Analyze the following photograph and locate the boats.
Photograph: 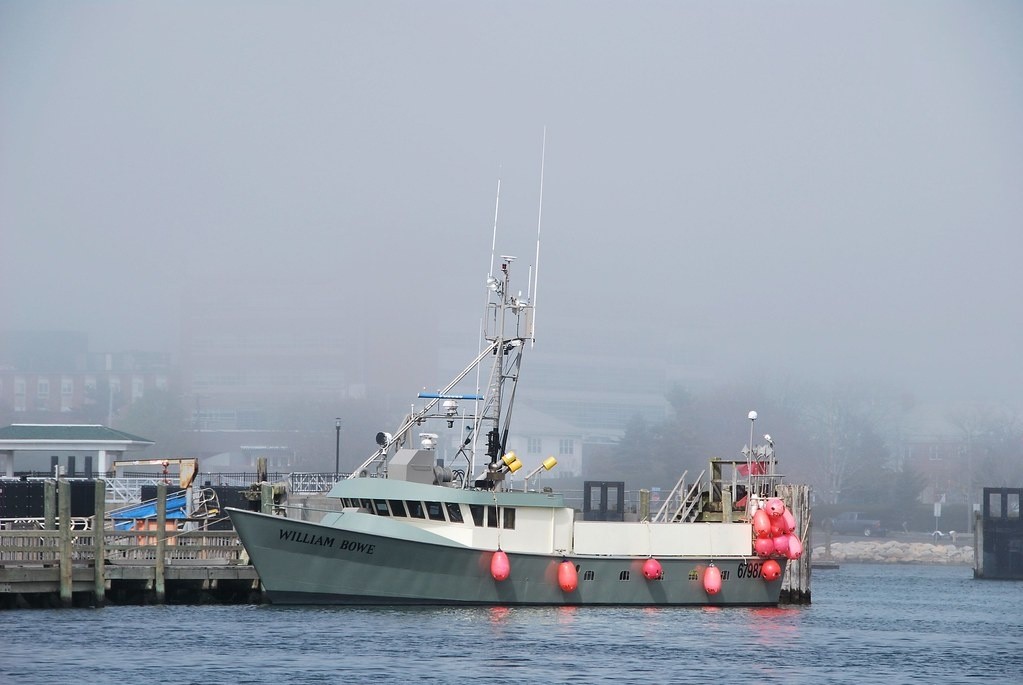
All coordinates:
[223,121,803,608]
[71,487,253,567]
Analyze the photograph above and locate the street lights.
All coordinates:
[335,416,342,483]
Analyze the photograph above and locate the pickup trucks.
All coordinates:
[821,510,887,537]
